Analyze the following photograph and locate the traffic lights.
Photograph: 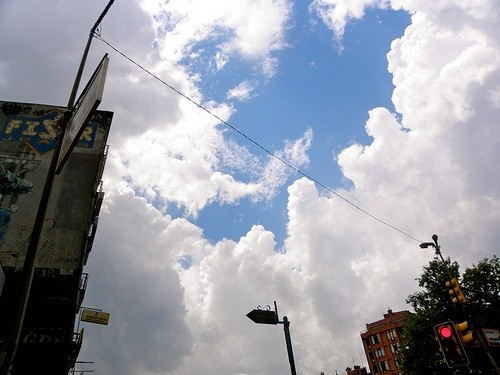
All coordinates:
[434,321,470,369]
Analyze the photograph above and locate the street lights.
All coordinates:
[419,233,499,375]
